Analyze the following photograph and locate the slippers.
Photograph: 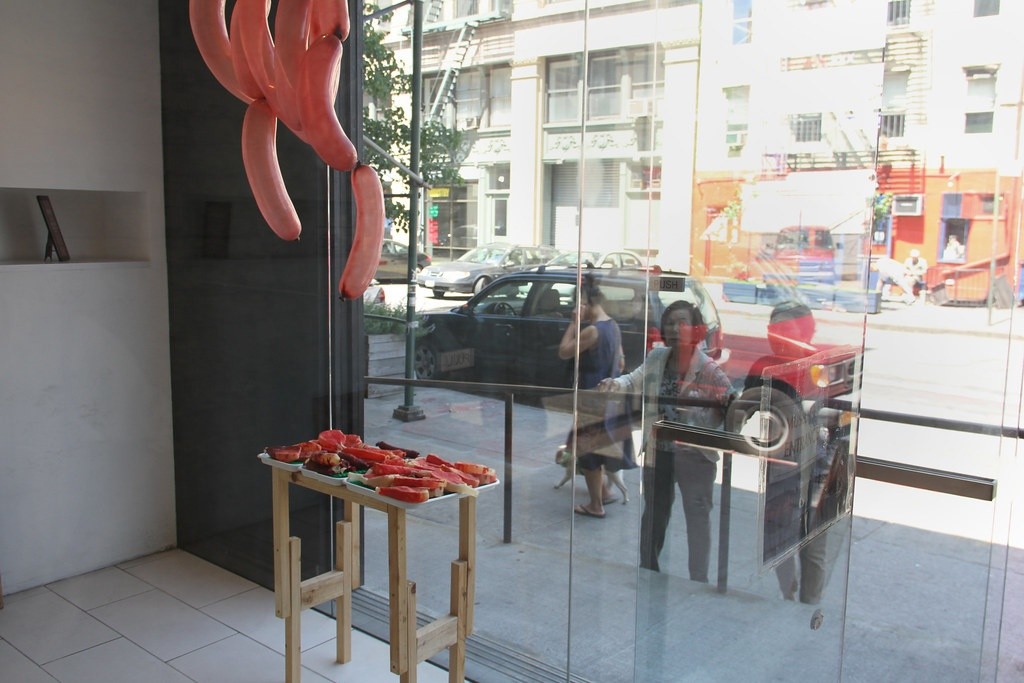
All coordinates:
[574,503,606,518]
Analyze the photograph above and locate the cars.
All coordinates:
[520,250,643,304]
[423,243,564,300]
[371,237,433,283]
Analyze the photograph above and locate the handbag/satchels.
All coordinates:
[571,383,615,420]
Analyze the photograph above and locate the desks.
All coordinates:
[268,454,479,683]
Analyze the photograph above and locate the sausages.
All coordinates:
[189,0,386,303]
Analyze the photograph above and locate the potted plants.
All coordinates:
[834,284,881,315]
[362,292,435,399]
[757,282,795,306]
[796,280,834,311]
[868,192,892,241]
[721,278,761,304]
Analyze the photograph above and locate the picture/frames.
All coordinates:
[35,194,69,262]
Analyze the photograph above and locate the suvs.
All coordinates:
[416,261,723,411]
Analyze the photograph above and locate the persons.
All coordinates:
[942,234,965,262]
[742,299,841,605]
[596,300,733,584]
[904,249,927,290]
[558,282,625,518]
